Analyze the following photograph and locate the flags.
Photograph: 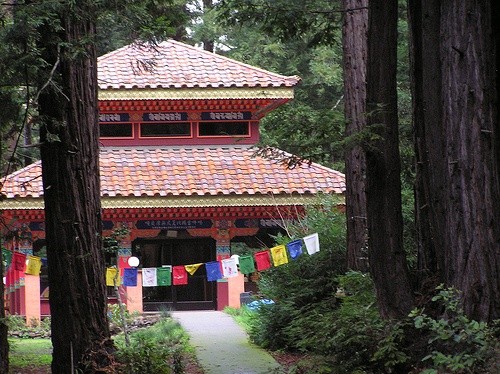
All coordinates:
[0,232,332,287]
